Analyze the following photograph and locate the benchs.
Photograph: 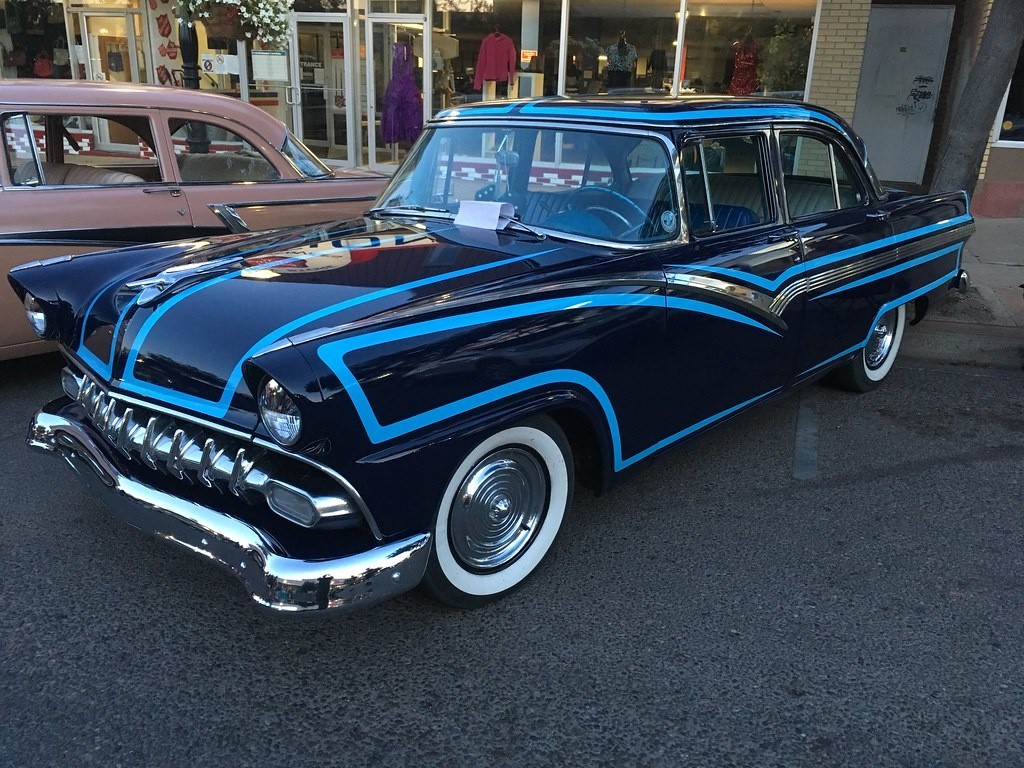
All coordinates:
[625,174,855,222]
[180,153,276,183]
[13,161,144,185]
[512,190,761,242]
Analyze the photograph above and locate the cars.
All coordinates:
[301,87,383,147]
[0,77,460,362]
[7,88,977,624]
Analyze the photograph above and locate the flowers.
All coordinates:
[171,0,295,53]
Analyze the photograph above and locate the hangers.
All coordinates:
[482,24,513,42]
